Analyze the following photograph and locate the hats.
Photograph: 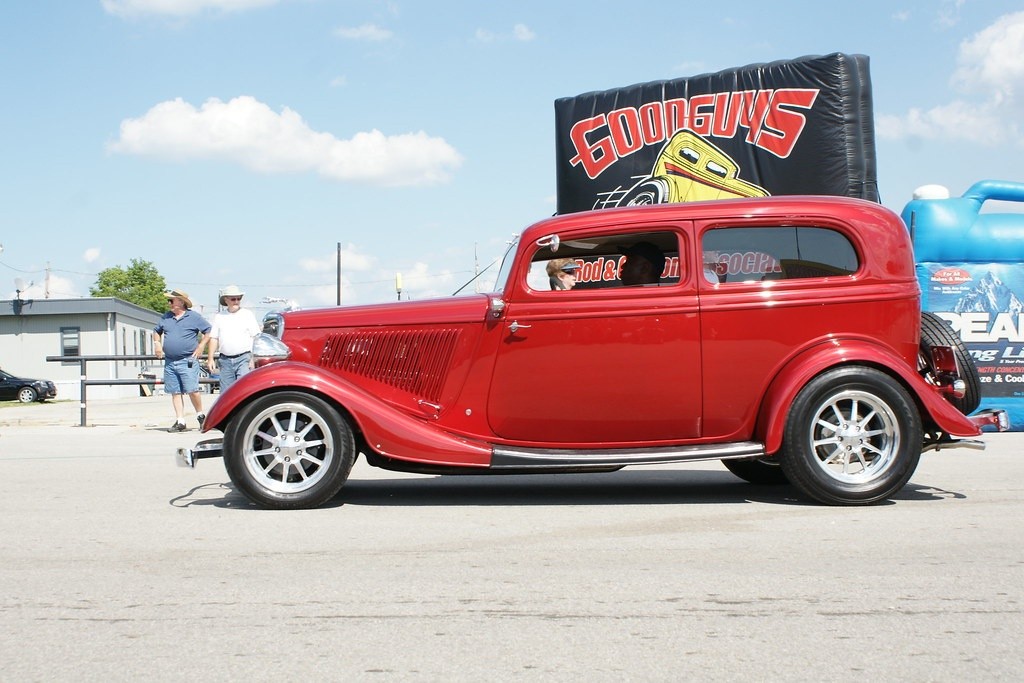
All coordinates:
[164,290,192,308]
[547,258,581,277]
[219,287,243,306]
[617,241,665,272]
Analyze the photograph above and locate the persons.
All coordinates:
[154,289,212,432]
[546,258,580,290]
[207,286,261,395]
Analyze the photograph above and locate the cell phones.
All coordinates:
[188,360,193,368]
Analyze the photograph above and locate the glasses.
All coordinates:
[167,298,182,304]
[556,269,575,275]
[226,297,241,302]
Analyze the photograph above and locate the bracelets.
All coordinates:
[154,341,160,343]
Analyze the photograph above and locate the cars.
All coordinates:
[176,193,1011,508]
[208,367,221,394]
[0,369,58,404]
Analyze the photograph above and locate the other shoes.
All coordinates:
[167,421,186,433]
[198,415,206,431]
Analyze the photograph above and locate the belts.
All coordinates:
[221,351,250,358]
